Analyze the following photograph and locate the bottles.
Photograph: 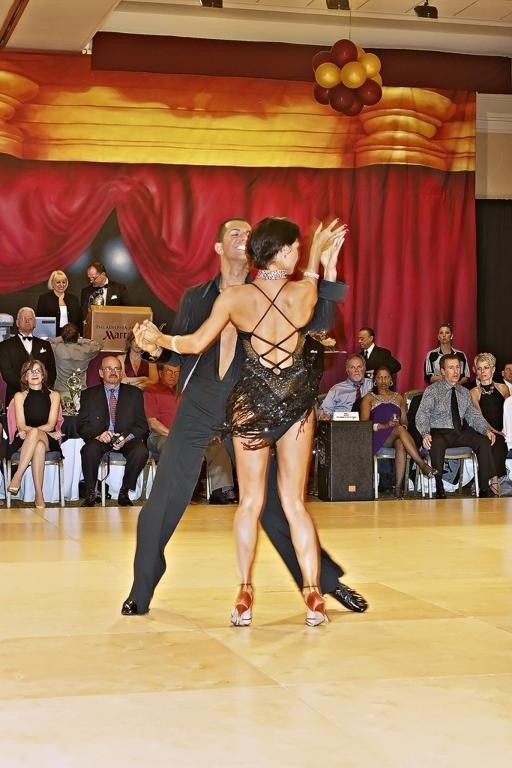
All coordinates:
[94,292,105,306]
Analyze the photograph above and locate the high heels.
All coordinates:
[7,478,21,494]
[229,580,255,629]
[34,493,47,511]
[419,461,440,480]
[298,582,331,629]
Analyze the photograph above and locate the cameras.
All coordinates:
[109,433,127,448]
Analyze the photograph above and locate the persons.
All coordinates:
[1,258,239,508]
[320,322,512,499]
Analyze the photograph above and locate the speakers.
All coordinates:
[309,420,373,501]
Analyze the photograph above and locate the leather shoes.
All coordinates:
[208,492,239,505]
[328,581,370,614]
[80,488,98,508]
[117,488,134,507]
[120,584,151,616]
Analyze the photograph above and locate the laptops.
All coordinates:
[34,317,56,337]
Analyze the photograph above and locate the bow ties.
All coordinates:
[22,335,34,342]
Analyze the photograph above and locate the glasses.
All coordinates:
[103,366,122,373]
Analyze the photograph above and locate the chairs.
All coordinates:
[0,394,217,507]
[304,382,484,497]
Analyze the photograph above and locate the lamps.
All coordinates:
[325,0,350,11]
[413,0,438,17]
[200,0,224,8]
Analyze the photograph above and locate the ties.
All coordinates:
[450,386,465,437]
